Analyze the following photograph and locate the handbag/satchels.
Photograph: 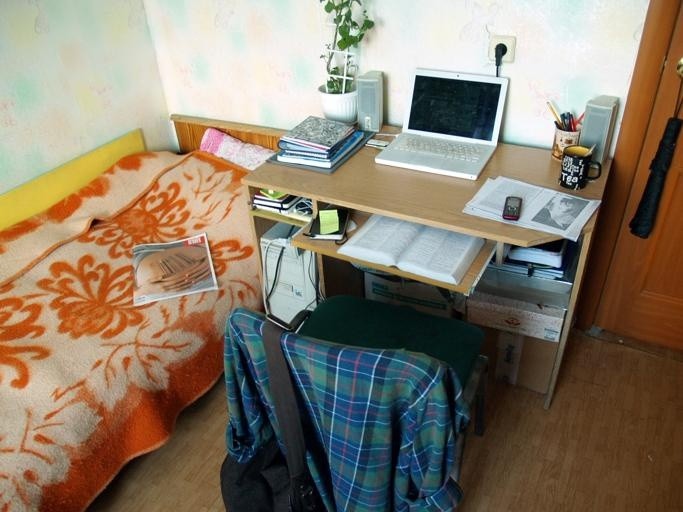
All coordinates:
[220,314,318,512]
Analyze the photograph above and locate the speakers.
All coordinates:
[579,95,620,168]
[356,71,384,133]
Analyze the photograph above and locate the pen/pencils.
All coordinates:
[545,101,584,132]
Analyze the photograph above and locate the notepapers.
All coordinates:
[318,210,338,234]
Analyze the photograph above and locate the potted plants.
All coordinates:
[319,0,374,124]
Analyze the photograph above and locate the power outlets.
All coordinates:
[488,36,516,63]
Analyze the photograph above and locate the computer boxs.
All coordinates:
[259,222,317,326]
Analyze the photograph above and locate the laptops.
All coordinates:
[375,69,509,181]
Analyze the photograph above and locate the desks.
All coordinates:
[240,125,613,411]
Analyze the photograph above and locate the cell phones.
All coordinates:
[503,196,522,220]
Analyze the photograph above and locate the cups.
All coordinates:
[558,145,602,191]
[551,123,582,162]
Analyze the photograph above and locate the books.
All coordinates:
[338,214,487,286]
[309,204,350,241]
[131,232,219,307]
[267,115,377,174]
[252,188,313,223]
[488,247,576,279]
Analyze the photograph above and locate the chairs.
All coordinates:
[226,295,489,483]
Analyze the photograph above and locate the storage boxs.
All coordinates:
[494,331,559,395]
[465,291,566,343]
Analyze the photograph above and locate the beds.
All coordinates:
[1,114,292,511]
